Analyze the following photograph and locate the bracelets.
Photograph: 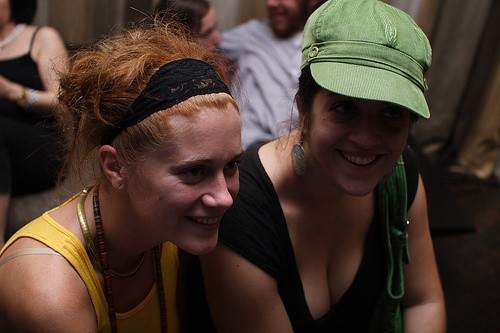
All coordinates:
[28,88,38,108]
[17,86,28,107]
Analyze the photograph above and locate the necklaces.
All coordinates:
[0,23,26,49]
[92,183,168,333]
[76,185,149,279]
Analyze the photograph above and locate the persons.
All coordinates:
[157,0,222,46]
[0,0,70,250]
[0,24,248,333]
[200,0,446,333]
[216,0,325,150]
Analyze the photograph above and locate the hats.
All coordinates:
[301,0,431,118]
